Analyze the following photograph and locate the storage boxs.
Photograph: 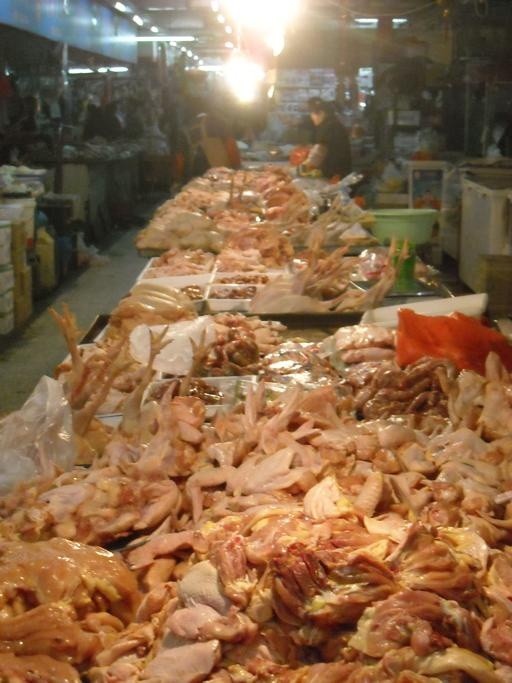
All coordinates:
[0,192,81,337]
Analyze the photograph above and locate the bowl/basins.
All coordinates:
[362,207,438,244]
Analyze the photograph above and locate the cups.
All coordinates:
[390,237,418,291]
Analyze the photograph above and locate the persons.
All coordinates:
[173,96,352,197]
